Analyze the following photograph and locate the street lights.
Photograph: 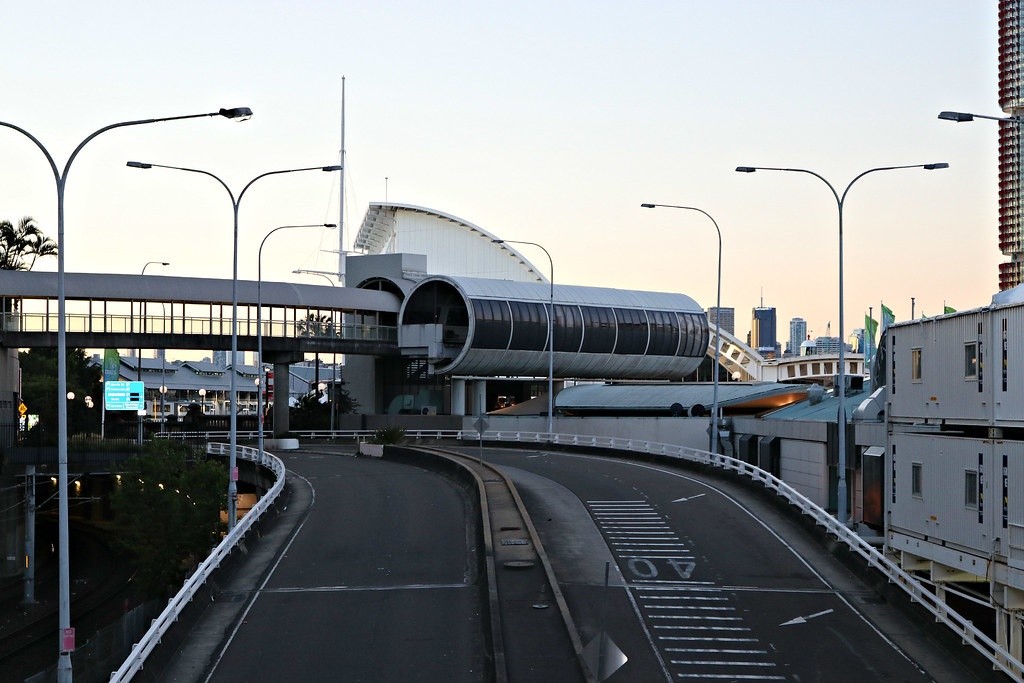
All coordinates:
[85,395,93,437]
[640,202,722,467]
[198,388,207,415]
[292,270,346,335]
[318,382,325,398]
[0,105,253,682]
[159,385,167,420]
[254,376,264,416]
[128,161,345,535]
[138,261,171,448]
[257,222,335,465]
[492,239,554,441]
[66,392,75,436]
[264,365,270,408]
[734,161,950,527]
